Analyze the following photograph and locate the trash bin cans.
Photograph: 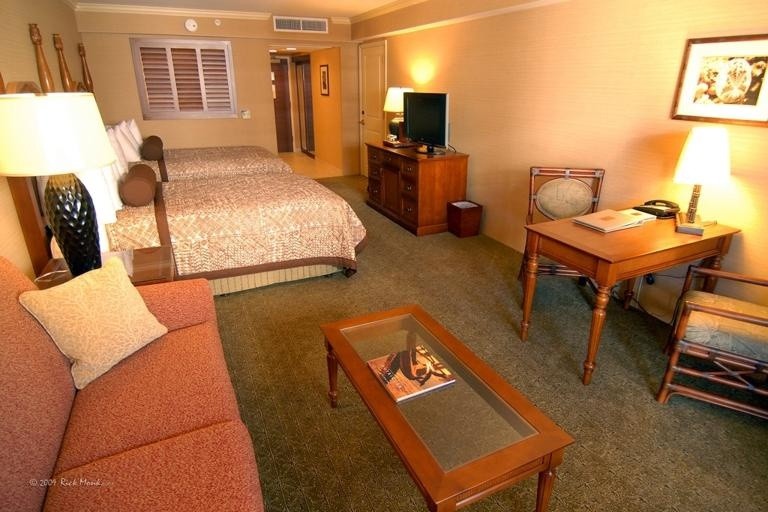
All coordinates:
[447,199,483,238]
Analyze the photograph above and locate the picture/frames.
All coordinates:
[319,64,330,97]
[667,33,768,132]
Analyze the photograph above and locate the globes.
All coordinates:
[524,177,593,276]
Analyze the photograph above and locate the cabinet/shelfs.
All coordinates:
[363,140,471,238]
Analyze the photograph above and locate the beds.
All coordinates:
[128,143,295,183]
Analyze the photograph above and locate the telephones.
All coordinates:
[633,199,680,217]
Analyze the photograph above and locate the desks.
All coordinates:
[519,208,742,388]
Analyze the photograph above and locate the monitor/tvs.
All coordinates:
[404,92,449,155]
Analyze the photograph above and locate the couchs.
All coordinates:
[0,250,267,511]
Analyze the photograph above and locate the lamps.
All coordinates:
[382,87,415,140]
[0,91,122,277]
[671,123,733,238]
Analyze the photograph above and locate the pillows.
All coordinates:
[15,255,171,395]
[72,117,165,256]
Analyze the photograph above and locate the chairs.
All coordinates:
[653,264,767,420]
[517,164,607,344]
[50,173,370,296]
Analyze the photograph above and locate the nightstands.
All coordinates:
[34,243,179,287]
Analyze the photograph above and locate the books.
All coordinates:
[620,209,657,223]
[367,344,456,404]
[573,207,643,233]
